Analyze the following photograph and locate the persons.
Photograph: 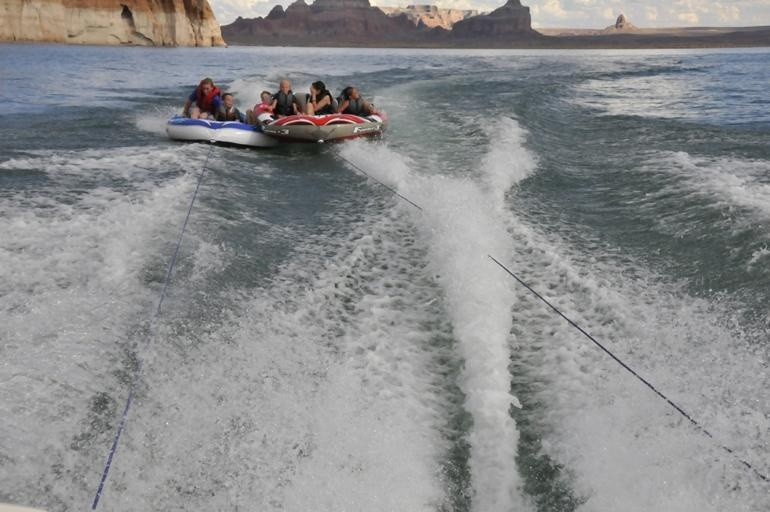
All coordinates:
[305,80,336,117]
[212,92,242,123]
[182,76,221,121]
[266,79,299,120]
[244,91,279,126]
[337,86,371,115]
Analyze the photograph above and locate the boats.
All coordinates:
[166,113,278,148]
[253,103,388,142]
